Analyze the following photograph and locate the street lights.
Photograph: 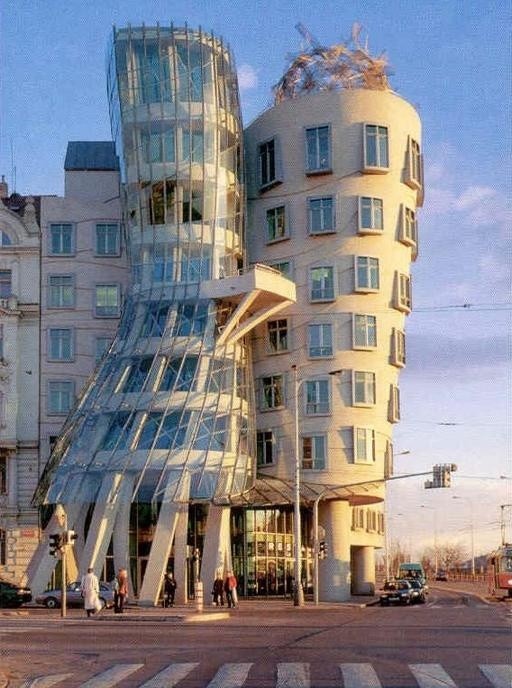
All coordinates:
[291,364,343,607]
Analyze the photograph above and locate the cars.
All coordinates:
[0,581,32,609]
[35,581,114,609]
[380,562,429,607]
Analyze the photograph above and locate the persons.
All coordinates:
[224,570,237,609]
[385,569,422,591]
[164,570,177,607]
[80,567,102,618]
[212,572,223,606]
[161,574,171,608]
[113,567,130,613]
